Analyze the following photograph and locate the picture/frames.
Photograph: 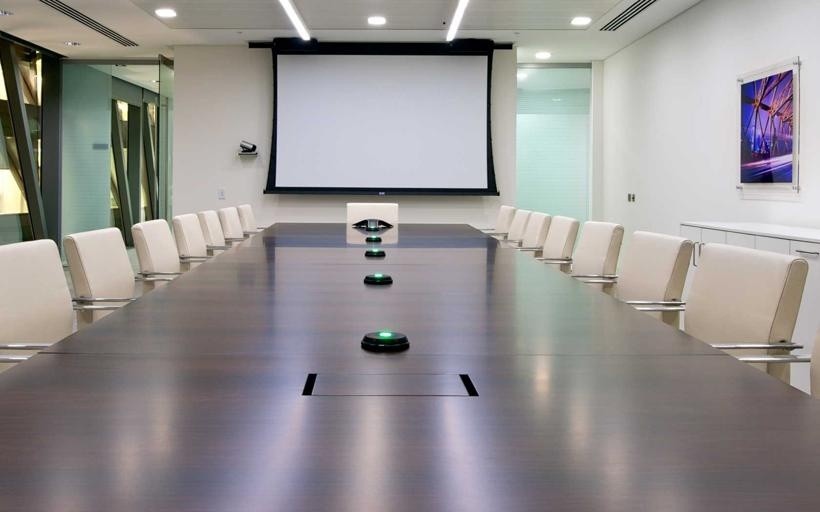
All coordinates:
[736,56,799,194]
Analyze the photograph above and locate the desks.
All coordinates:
[0,223,819,511]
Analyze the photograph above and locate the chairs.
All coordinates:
[63,227,172,330]
[516,216,579,273]
[133,218,208,295]
[478,205,516,238]
[740,306,820,397]
[1,238,121,357]
[171,212,227,271]
[238,203,268,236]
[219,206,256,246]
[499,212,551,257]
[487,209,530,246]
[345,202,399,222]
[543,221,626,298]
[196,209,243,256]
[638,241,809,384]
[582,229,695,330]
